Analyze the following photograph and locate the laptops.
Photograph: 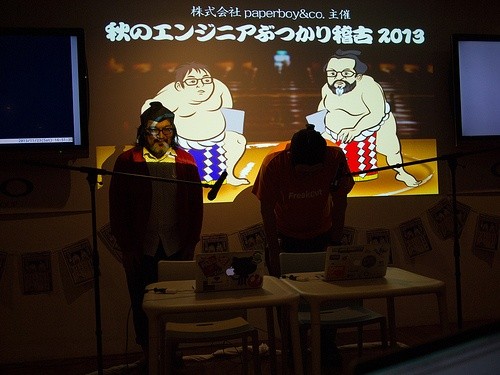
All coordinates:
[315,245,388,282]
[190,250,265,293]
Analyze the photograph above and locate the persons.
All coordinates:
[108,103,204,357]
[250,125,355,275]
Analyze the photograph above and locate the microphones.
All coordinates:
[207,171,228,201]
[330,157,349,193]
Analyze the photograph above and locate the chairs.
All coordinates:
[280,251,387,372]
[160,259,262,375]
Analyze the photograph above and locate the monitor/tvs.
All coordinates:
[0,27,89,151]
[448,32,500,145]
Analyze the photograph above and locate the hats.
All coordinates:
[290,123,328,165]
[141,101,174,123]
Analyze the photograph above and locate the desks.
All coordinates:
[142,275,303,375]
[280,266,445,375]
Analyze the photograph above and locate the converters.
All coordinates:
[296,276,309,281]
[165,288,177,294]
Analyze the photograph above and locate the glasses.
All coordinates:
[143,126,174,137]
[180,75,213,86]
[326,69,356,78]
